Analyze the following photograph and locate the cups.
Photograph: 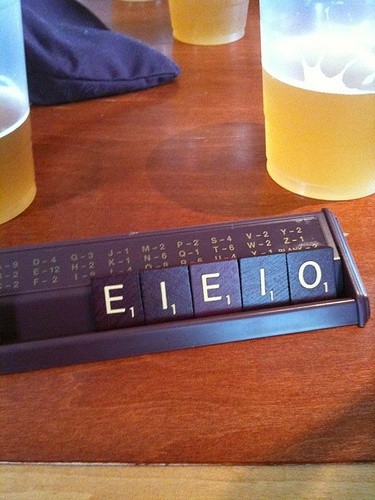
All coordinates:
[257,0,375,201]
[1,0,39,225]
[167,0,252,46]
